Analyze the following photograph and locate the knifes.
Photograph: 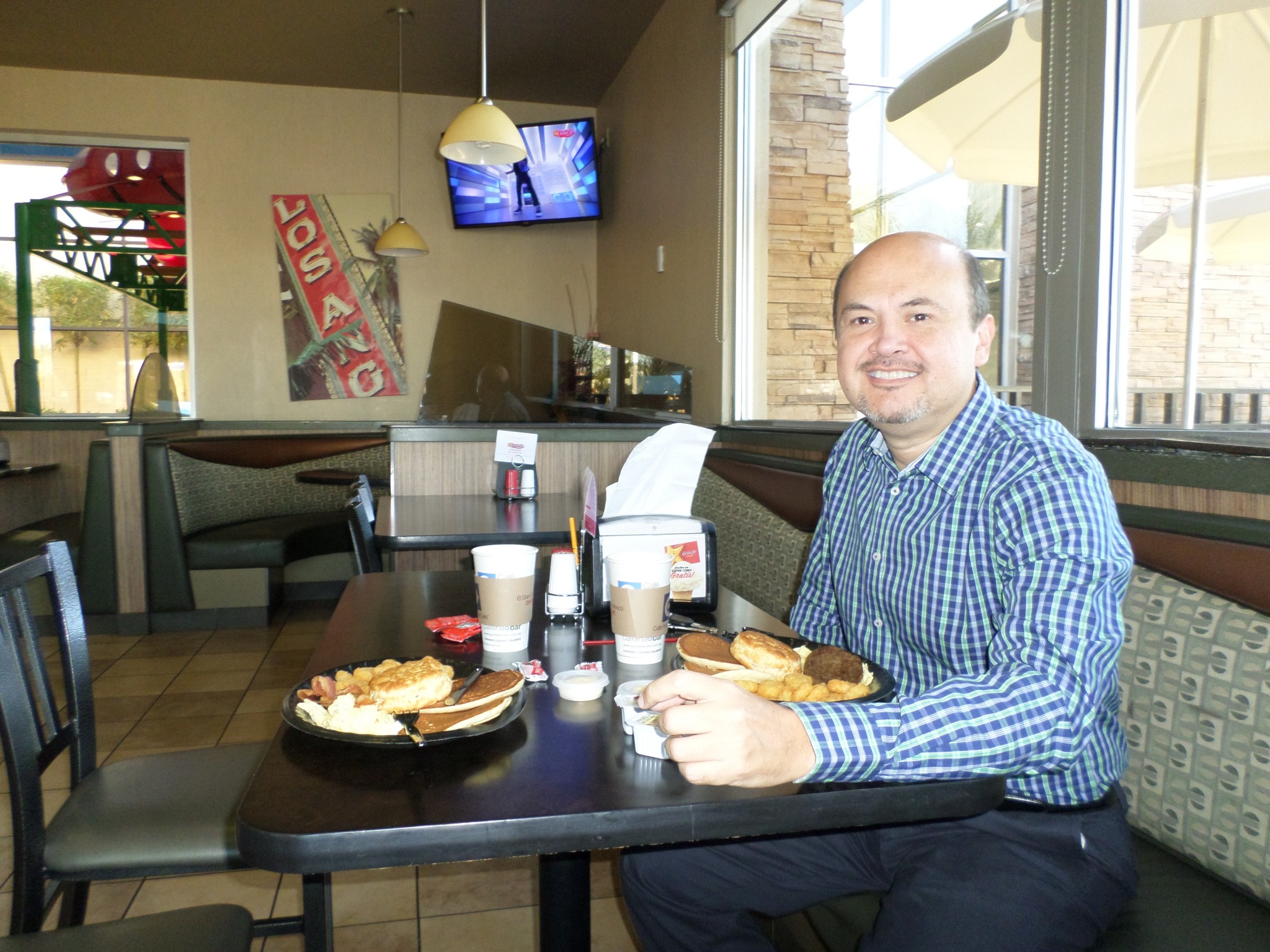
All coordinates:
[444,667,483,705]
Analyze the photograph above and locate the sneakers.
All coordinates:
[513,208,523,215]
[535,206,542,217]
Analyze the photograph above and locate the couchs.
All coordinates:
[145,431,391,633]
[0,436,118,617]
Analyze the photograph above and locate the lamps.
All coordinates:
[437,0,528,166]
[372,8,429,259]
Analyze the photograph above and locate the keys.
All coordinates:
[665,612,719,633]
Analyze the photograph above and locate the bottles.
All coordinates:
[505,470,518,498]
[548,547,578,615]
[520,470,534,497]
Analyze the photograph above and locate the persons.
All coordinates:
[475,364,532,422]
[505,157,542,218]
[617,228,1138,952]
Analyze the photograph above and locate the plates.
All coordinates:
[671,636,896,703]
[280,656,528,747]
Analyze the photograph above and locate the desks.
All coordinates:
[0,462,58,483]
[372,492,602,570]
[293,466,392,532]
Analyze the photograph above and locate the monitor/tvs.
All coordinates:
[441,118,603,229]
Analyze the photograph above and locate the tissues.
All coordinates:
[582,423,720,617]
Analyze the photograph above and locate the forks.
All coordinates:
[393,710,428,746]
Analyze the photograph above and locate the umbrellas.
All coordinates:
[881,0,1270,432]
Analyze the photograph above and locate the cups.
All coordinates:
[602,551,675,664]
[471,545,539,652]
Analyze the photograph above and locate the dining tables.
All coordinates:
[234,569,1010,952]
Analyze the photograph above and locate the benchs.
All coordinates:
[688,444,1270,951]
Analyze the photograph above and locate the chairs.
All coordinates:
[0,627,257,952]
[346,474,377,527]
[0,539,336,952]
[338,490,381,575]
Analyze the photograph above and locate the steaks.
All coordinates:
[803,644,864,686]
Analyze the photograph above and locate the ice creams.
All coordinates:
[669,563,702,601]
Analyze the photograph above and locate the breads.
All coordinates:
[369,656,525,735]
[675,631,803,683]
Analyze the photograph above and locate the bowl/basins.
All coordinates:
[552,670,610,701]
[616,680,656,735]
